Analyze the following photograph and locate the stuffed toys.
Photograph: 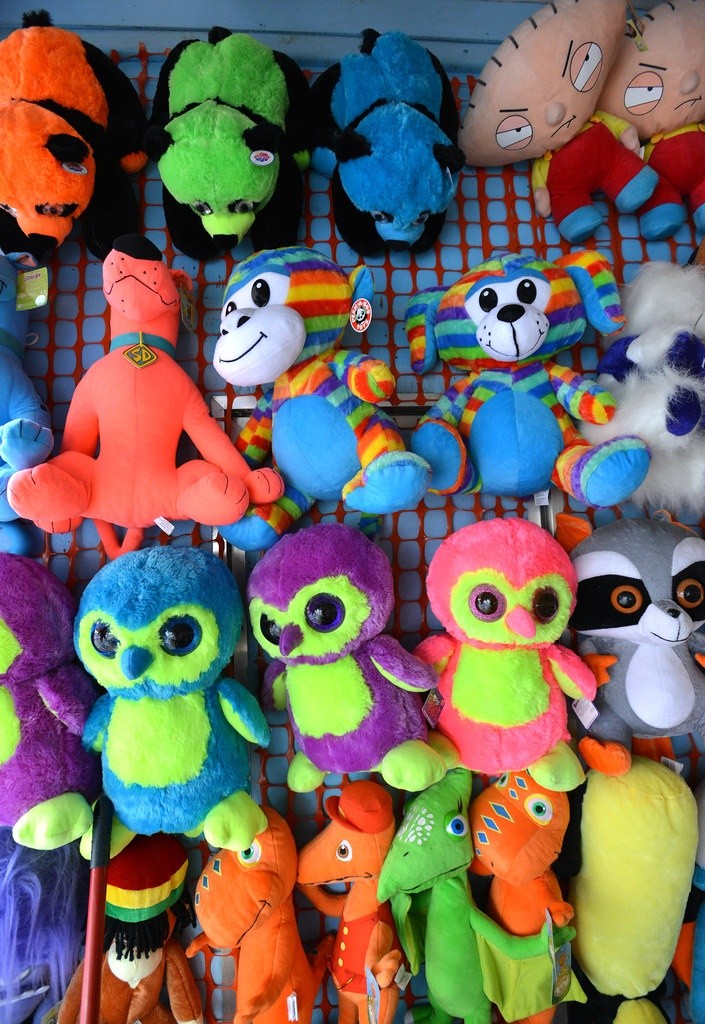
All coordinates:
[552,507,704,778]
[453,0,661,247]
[413,515,601,788]
[574,258,705,527]
[307,28,469,259]
[210,247,437,554]
[6,231,287,565]
[0,753,705,1024]
[403,252,654,509]
[68,541,276,860]
[598,0,704,239]
[144,22,321,263]
[0,8,156,260]
[241,521,448,798]
[1,551,96,854]
[0,246,53,555]
